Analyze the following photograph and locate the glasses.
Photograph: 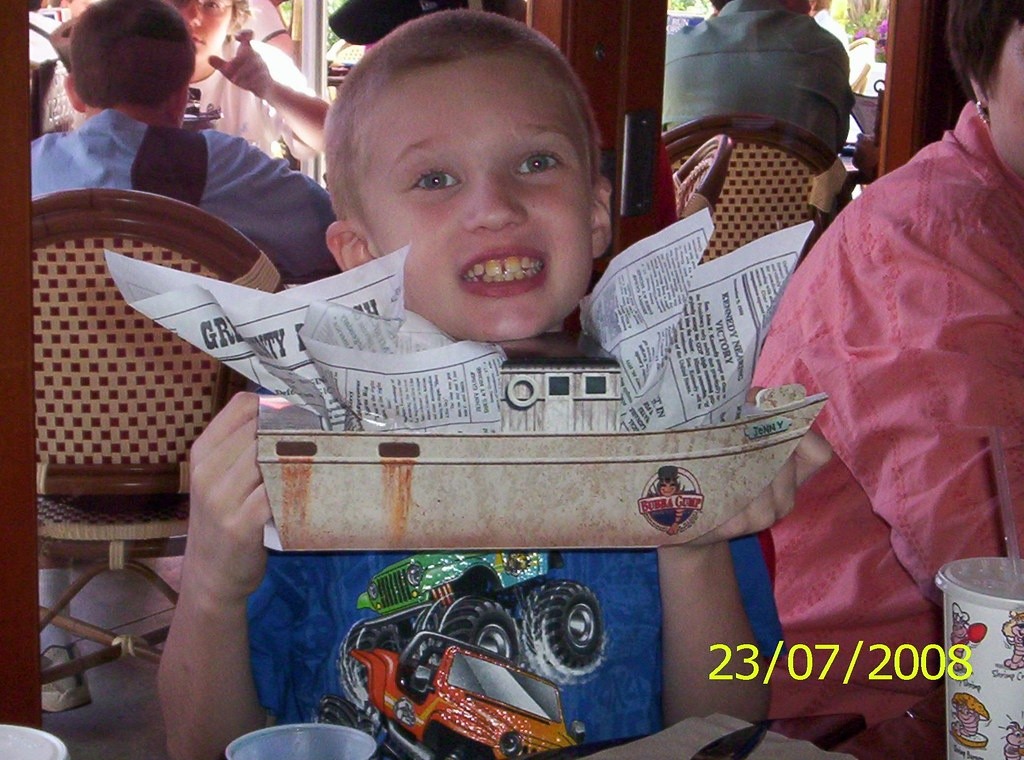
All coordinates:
[175,0,232,16]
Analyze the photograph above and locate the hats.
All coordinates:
[328,0,513,45]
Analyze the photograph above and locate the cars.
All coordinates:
[324,38,366,87]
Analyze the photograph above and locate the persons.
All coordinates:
[27,1,103,141]
[741,0,1022,759]
[154,9,790,759]
[661,1,856,157]
[807,0,850,55]
[241,0,296,58]
[31,0,344,713]
[327,0,510,61]
[156,0,334,164]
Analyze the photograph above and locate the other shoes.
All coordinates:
[40,643,90,714]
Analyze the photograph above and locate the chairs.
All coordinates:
[664,114,856,267]
[30,185,286,662]
